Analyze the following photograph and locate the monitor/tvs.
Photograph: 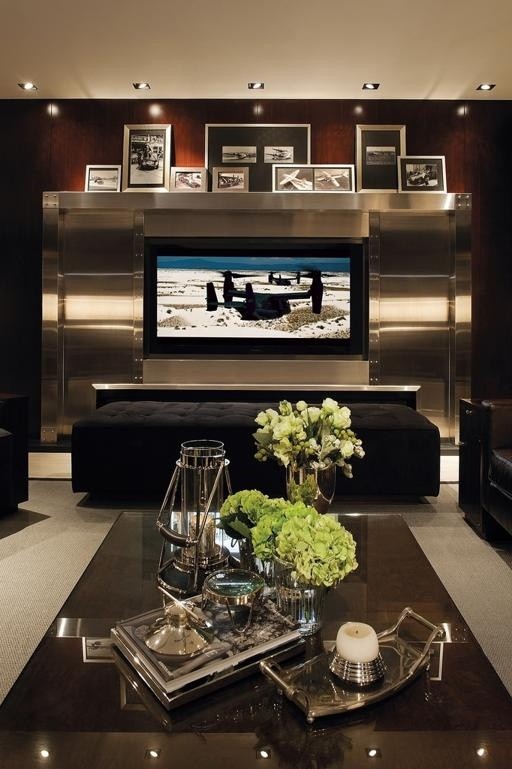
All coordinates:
[142,237,369,361]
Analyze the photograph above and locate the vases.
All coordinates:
[238,539,321,634]
[284,462,338,515]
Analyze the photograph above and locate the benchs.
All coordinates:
[73,400,441,503]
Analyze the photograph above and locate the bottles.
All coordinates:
[169,436,231,574]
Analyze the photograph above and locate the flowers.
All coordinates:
[251,399,364,473]
[210,486,360,586]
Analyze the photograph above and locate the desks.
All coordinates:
[88,382,420,409]
[2,507,512,769]
